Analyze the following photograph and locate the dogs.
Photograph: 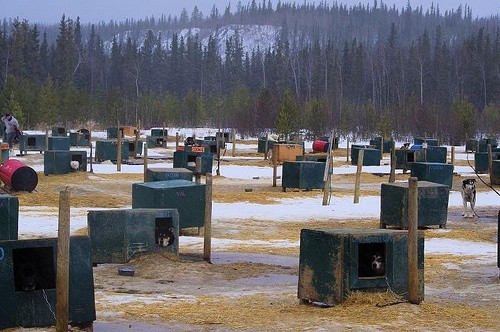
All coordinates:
[154,226,175,247]
[70,160,80,169]
[368,253,386,276]
[15,269,42,292]
[461,179,476,218]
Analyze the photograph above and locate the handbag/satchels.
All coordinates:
[14,125,21,138]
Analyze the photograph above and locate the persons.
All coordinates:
[0,113,21,150]
